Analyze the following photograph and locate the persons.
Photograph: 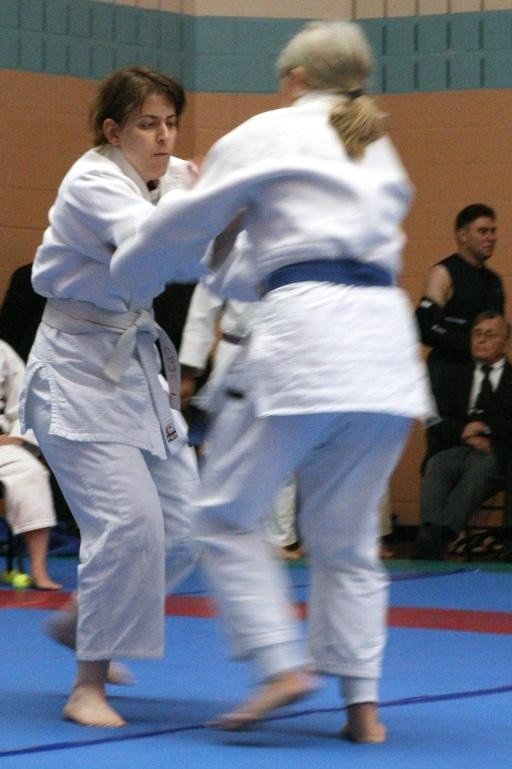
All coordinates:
[18,64,250,730]
[413,203,510,474]
[108,11,440,747]
[1,326,61,594]
[398,307,512,563]
[178,224,306,562]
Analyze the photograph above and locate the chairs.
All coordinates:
[447,473,512,564]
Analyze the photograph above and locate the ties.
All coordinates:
[474,364,494,414]
